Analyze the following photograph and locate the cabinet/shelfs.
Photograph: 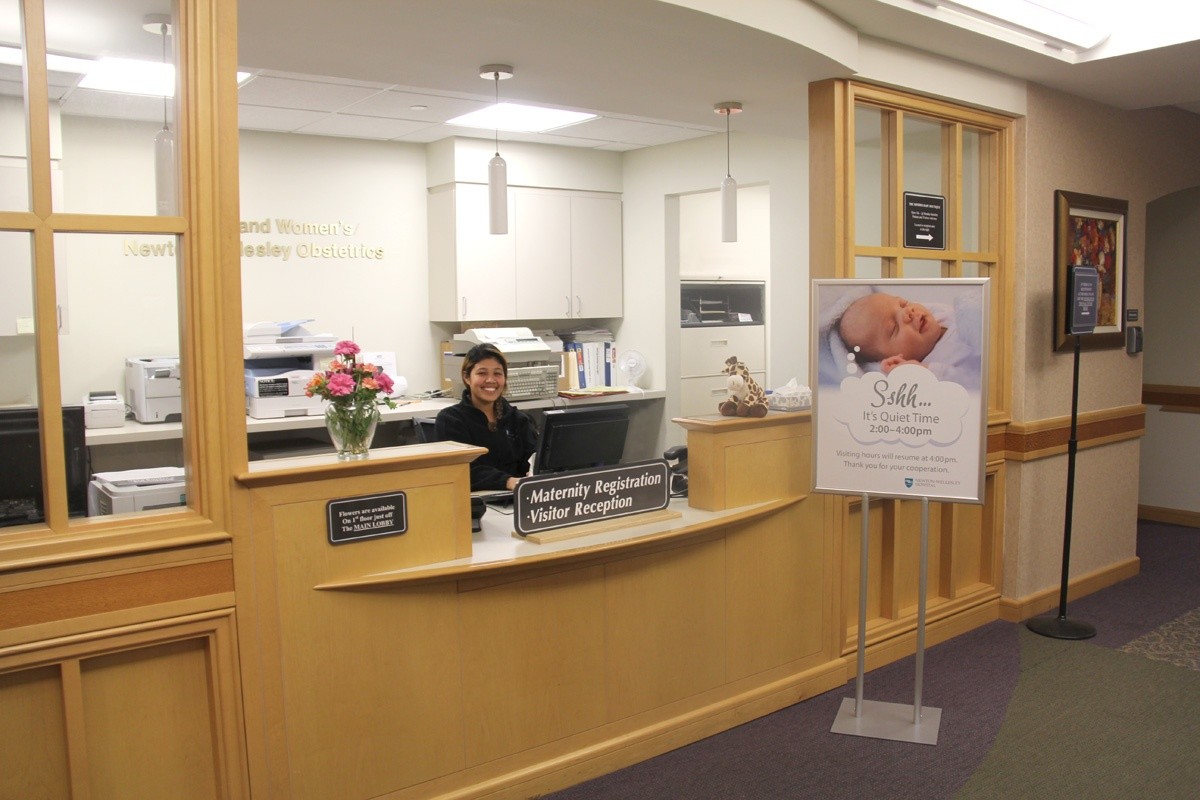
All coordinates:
[675,185,770,453]
[423,135,627,326]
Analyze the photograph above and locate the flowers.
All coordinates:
[305,339,398,454]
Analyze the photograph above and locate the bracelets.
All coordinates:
[513,478,521,490]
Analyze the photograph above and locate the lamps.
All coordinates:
[477,61,518,237]
[711,101,746,246]
[141,12,186,222]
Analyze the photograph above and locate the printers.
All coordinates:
[84,390,124,429]
[124,354,182,425]
[244,335,341,420]
[88,466,187,520]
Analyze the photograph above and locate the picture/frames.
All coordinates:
[1053,187,1129,354]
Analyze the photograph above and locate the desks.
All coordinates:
[84,388,671,483]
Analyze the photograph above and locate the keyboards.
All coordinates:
[480,490,513,508]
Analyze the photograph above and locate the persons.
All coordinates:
[435,342,542,491]
[839,292,982,396]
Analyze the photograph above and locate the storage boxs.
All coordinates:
[765,390,814,412]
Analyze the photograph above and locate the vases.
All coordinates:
[322,400,380,459]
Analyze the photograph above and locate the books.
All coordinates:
[559,325,629,398]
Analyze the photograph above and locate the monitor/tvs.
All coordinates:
[532,403,630,477]
